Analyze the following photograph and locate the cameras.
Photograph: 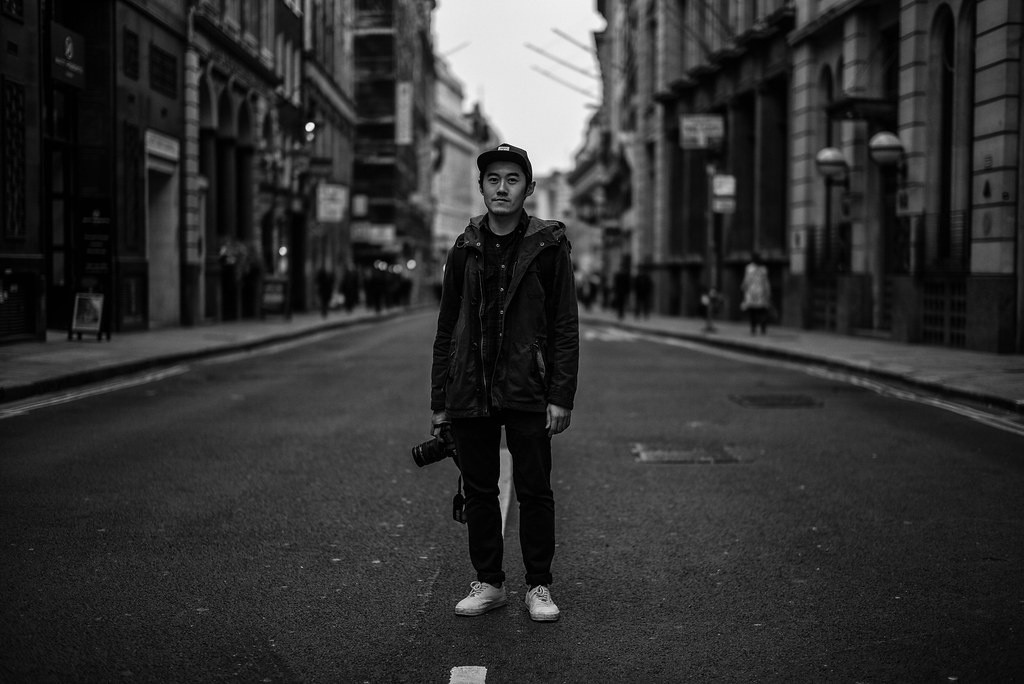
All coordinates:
[412,426,456,467]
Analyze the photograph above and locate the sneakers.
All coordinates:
[525,585,560,621]
[454,581,506,616]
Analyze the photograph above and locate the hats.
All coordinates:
[476,143,532,174]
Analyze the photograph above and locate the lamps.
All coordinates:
[815,147,851,187]
[868,131,909,178]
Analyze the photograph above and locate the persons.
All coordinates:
[576,263,653,319]
[312,256,414,319]
[739,251,772,334]
[429,142,581,620]
